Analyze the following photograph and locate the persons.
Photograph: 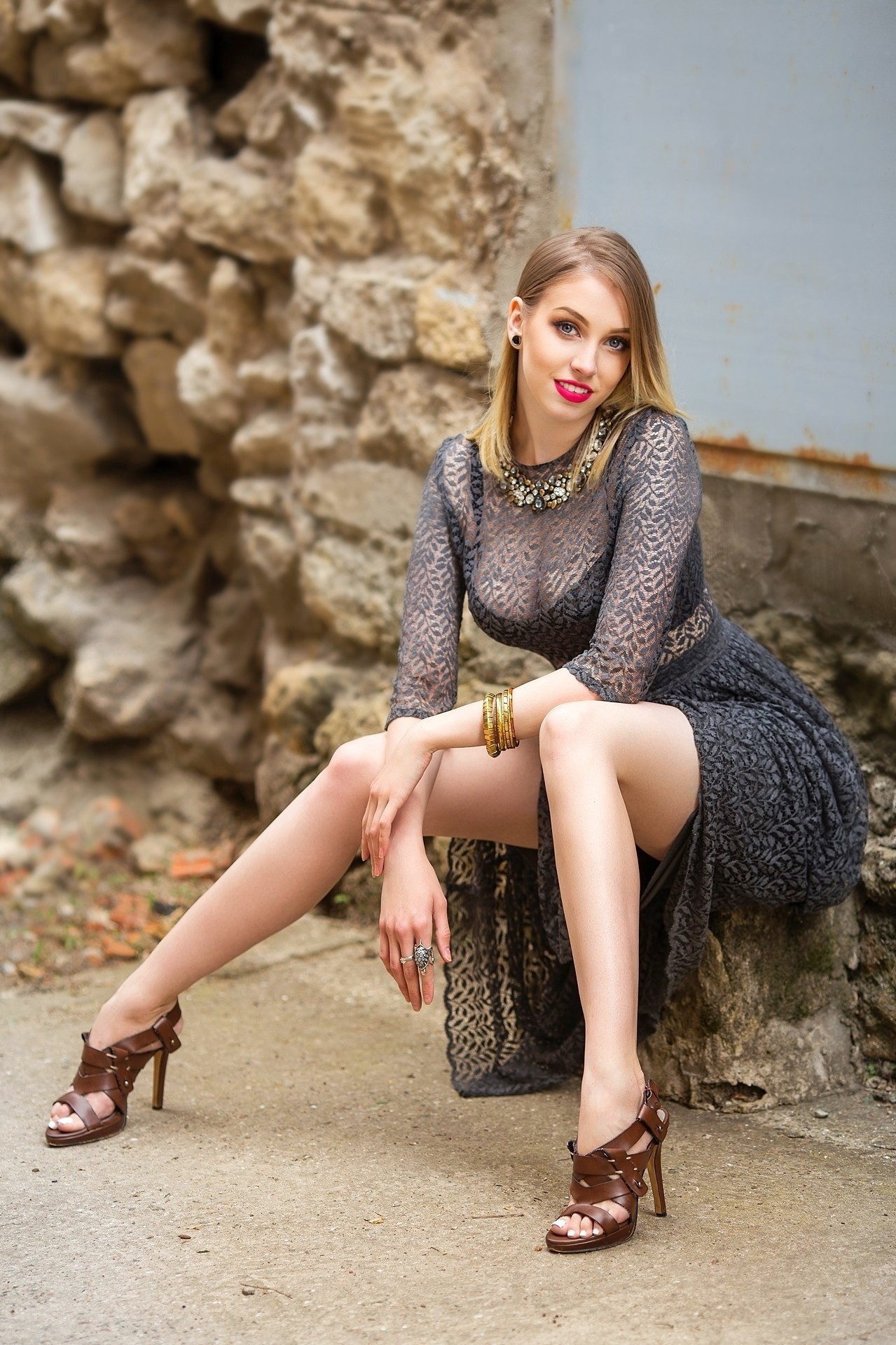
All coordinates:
[42,228,866,1257]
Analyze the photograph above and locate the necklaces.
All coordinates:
[497,403,613,515]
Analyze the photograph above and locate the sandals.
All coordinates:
[546,1078,670,1253]
[46,997,182,1146]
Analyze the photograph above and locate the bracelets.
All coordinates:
[482,688,520,758]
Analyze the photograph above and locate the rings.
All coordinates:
[400,954,415,964]
[414,939,434,976]
